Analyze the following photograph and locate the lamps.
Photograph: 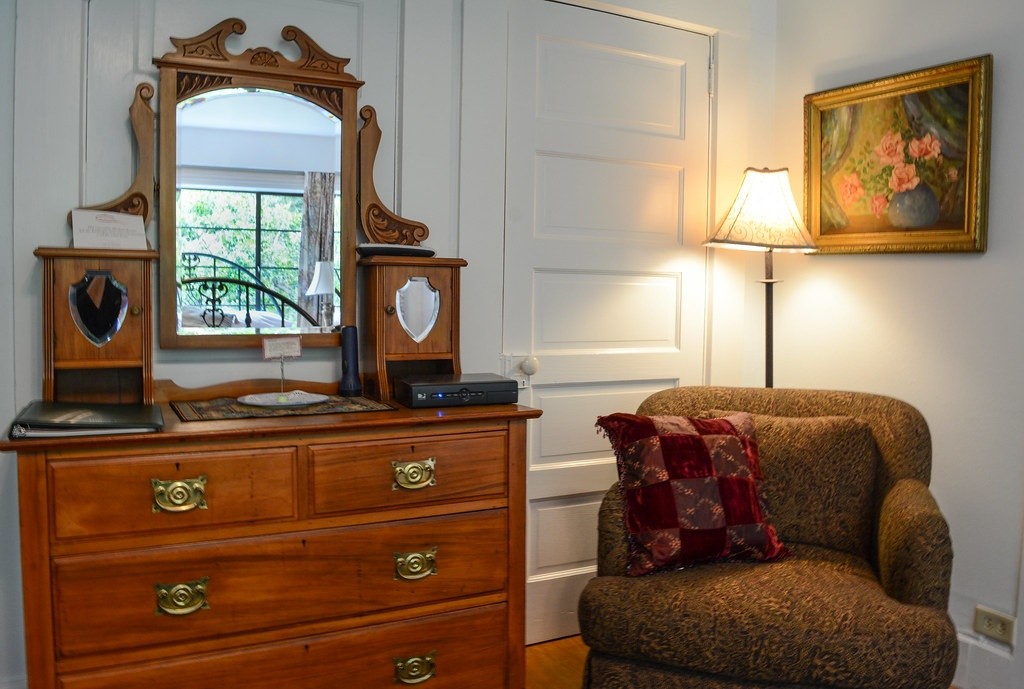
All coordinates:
[702,167,822,389]
[306,260,335,333]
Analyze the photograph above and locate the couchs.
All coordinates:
[578,386,959,689]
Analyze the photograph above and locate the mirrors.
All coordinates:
[75,17,430,404]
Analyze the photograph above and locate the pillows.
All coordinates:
[181,305,240,327]
[596,410,795,576]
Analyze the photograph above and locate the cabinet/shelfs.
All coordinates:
[0,397,543,689]
[29,244,159,409]
[353,254,469,401]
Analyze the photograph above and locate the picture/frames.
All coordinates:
[803,54,993,256]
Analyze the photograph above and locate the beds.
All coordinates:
[176,252,322,328]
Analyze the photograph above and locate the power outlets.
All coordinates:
[973,602,1021,647]
